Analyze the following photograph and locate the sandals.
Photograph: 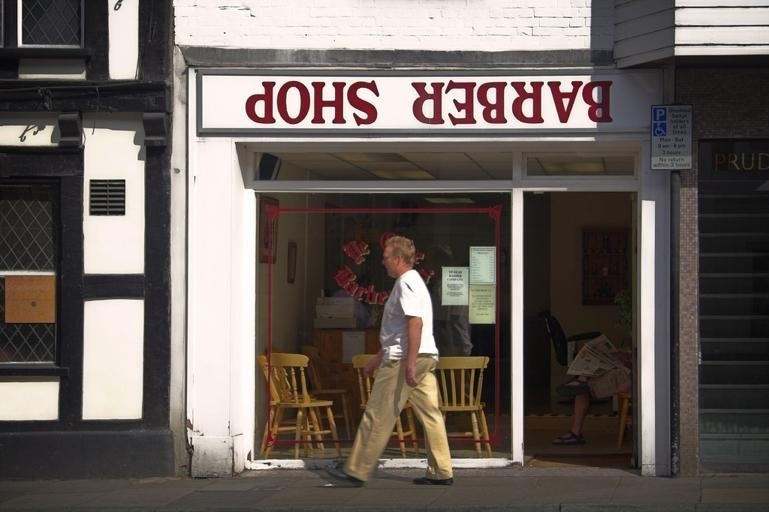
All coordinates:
[552,431,586,446]
[556,378,590,396]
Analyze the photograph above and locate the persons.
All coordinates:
[421,242,473,358]
[551,349,633,446]
[324,234,454,486]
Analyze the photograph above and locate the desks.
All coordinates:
[309,328,381,428]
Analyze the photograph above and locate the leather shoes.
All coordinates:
[326,468,365,486]
[413,475,454,485]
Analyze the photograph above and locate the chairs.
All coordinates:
[256,342,356,461]
[348,354,492,458]
[545,314,611,406]
